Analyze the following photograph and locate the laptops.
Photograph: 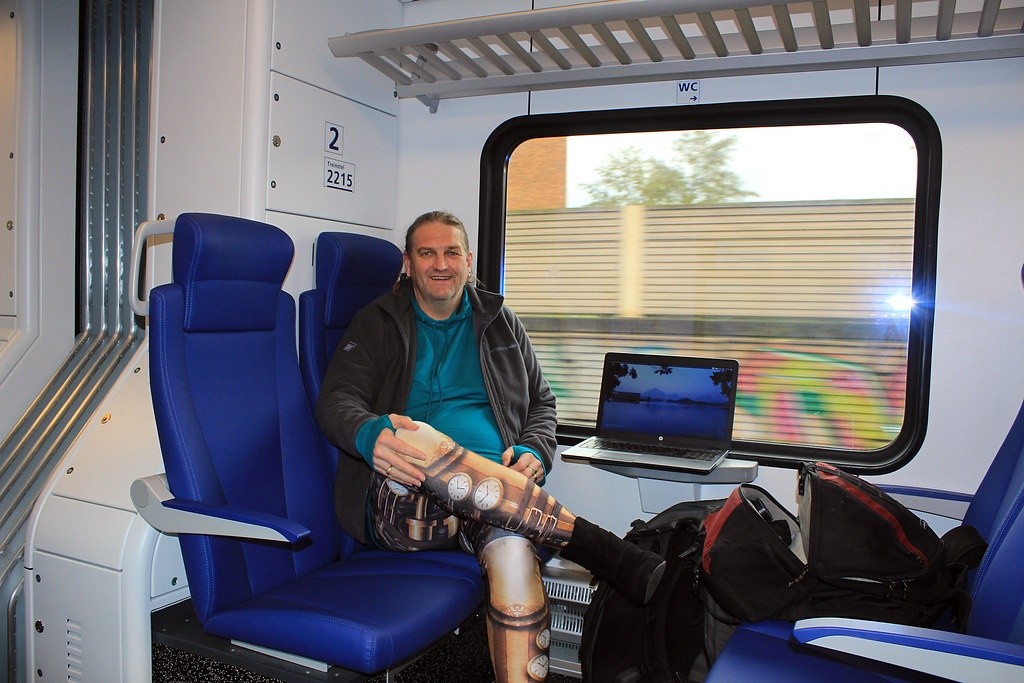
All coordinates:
[560,352,740,475]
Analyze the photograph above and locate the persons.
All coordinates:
[312,211,671,683]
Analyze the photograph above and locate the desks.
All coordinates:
[589,458,758,484]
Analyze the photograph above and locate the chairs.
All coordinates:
[130,212,561,683]
[703,399,1023,683]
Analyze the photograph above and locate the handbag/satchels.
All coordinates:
[701,462,943,625]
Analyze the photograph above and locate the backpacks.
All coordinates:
[578,497,730,683]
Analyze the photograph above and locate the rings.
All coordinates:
[528,465,539,476]
[386,465,393,474]
[532,474,539,483]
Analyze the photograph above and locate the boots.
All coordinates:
[560,517,667,603]
